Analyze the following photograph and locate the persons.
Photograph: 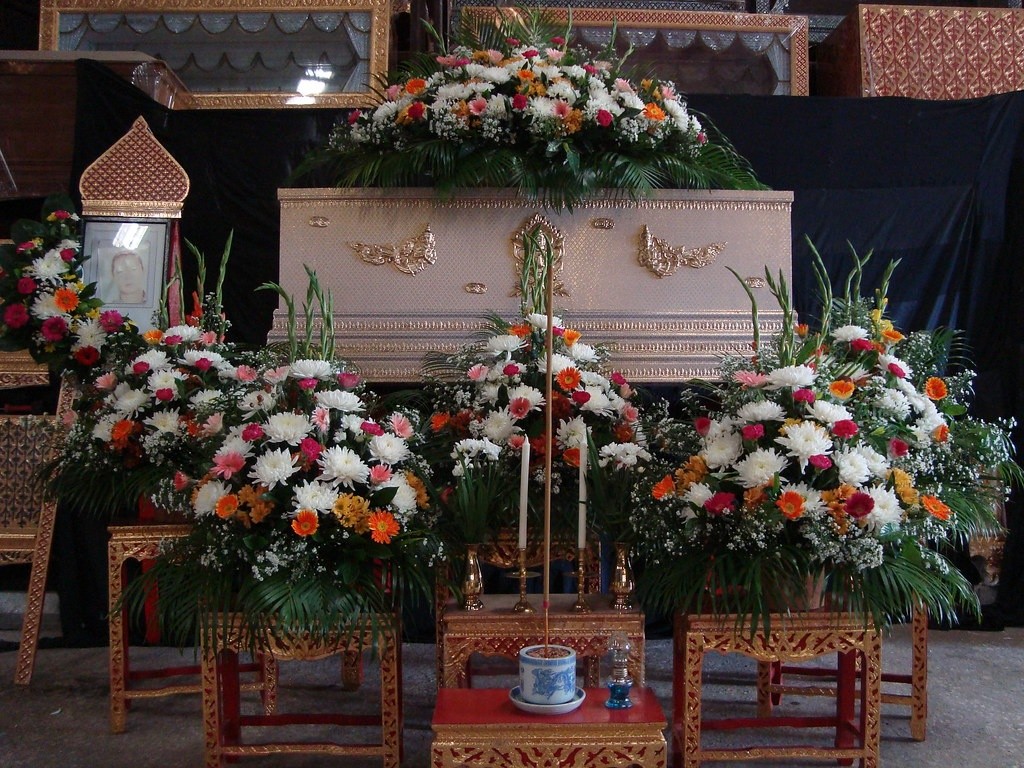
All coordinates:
[108,250,146,305]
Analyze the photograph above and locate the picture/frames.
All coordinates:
[74,216,173,333]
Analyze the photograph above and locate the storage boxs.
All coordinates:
[810,3,1024,100]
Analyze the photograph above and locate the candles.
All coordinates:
[578,427,588,549]
[518,433,532,549]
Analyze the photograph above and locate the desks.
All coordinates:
[105,524,281,735]
[431,686,669,768]
[672,611,885,768]
[199,612,405,768]
[435,526,603,691]
[443,593,646,687]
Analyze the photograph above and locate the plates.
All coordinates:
[509,685,586,715]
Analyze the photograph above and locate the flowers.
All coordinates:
[0,0,1024,645]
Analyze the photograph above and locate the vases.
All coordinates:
[518,644,578,704]
[769,566,825,610]
[757,577,929,743]
